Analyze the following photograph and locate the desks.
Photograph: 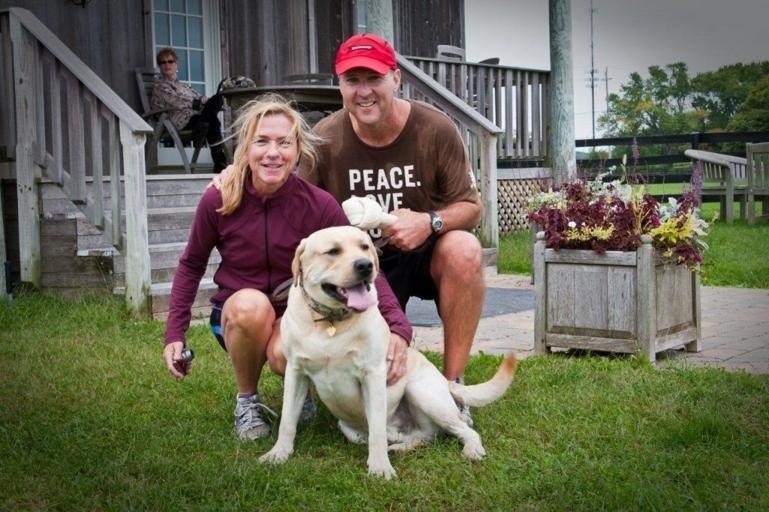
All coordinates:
[217,85,343,167]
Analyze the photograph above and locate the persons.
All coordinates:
[150,47,228,174]
[164,92,413,444]
[297,33,486,432]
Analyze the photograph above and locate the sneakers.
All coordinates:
[299,390,315,426]
[235,392,278,443]
[446,378,474,436]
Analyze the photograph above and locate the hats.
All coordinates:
[335,33,396,75]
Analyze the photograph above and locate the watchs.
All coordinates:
[427,209,443,236]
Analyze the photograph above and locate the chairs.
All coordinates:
[479,57,500,65]
[284,73,332,86]
[686,149,764,224]
[744,142,769,225]
[436,45,464,62]
[137,68,207,174]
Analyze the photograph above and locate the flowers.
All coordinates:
[526,171,707,273]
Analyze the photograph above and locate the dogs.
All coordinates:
[259,225,517,481]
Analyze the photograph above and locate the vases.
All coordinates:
[533,231,703,367]
